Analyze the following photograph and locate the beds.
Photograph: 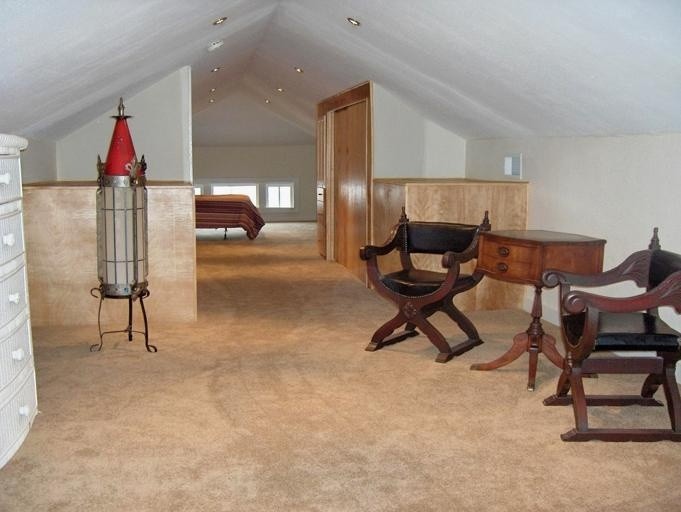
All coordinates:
[195,193,264,240]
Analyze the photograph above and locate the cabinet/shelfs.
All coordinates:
[0,134,38,478]
[372,178,531,314]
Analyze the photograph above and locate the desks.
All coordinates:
[472,229,606,392]
[93,288,159,354]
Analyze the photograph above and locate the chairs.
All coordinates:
[543,227,680,444]
[353,202,489,363]
